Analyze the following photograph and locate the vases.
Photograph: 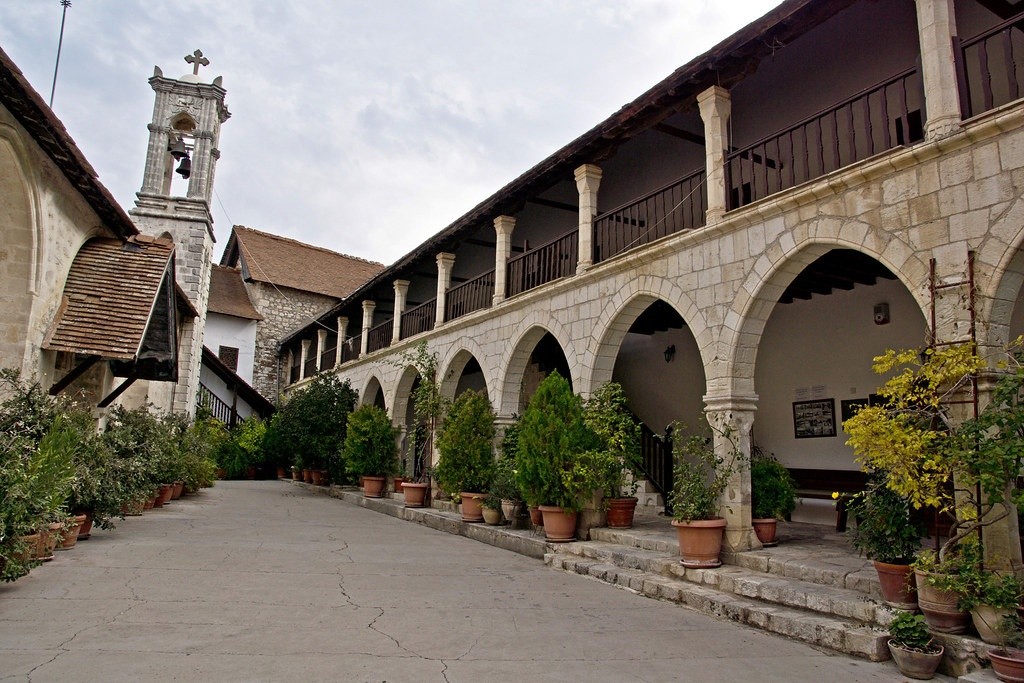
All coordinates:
[875,558,919,610]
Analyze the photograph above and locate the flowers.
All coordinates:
[832,467,922,563]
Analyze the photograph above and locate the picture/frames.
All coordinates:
[841,398,868,430]
[869,393,892,420]
[792,398,836,439]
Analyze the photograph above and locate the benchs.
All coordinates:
[781,467,874,533]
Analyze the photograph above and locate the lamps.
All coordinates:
[664,344,676,363]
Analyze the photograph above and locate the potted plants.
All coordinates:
[0,365,272,586]
[273,367,803,569]
[840,335,1024,683]
